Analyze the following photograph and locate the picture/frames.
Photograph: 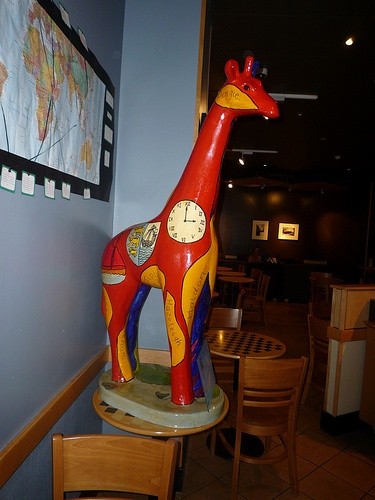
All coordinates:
[277,222,300,240]
[251,220,269,240]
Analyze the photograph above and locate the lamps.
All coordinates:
[266,91,320,101]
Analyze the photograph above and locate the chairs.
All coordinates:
[52,433,178,500]
[205,264,344,500]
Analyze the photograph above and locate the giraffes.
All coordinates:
[100,55,279,405]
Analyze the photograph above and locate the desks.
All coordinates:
[217,272,245,306]
[93,389,229,468]
[215,267,233,271]
[204,330,286,397]
[218,277,255,309]
